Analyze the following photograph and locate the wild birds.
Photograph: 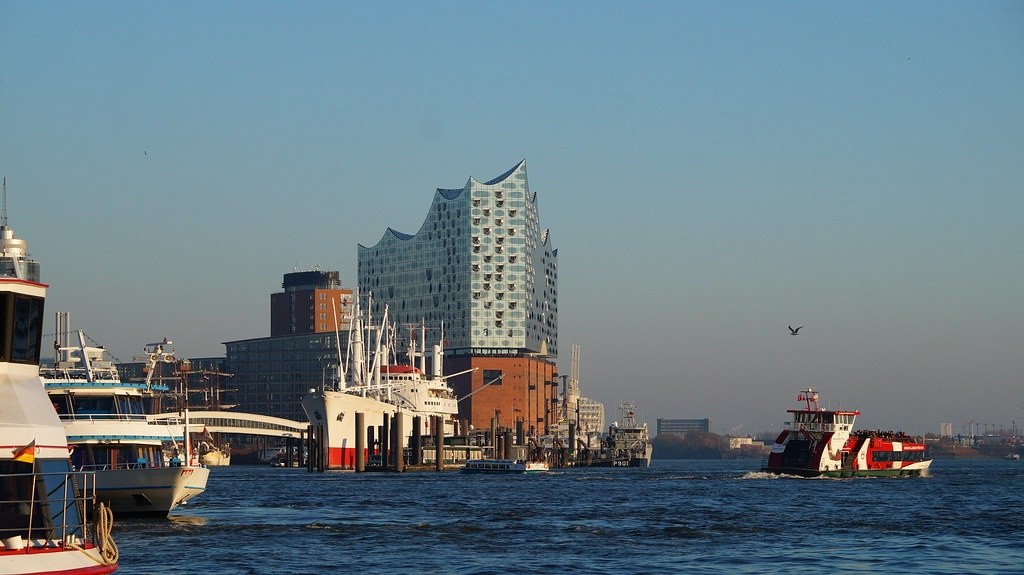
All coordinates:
[789,326,804,335]
[143,151,147,155]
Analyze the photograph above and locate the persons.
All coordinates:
[825,465,829,470]
[868,463,872,469]
[517,458,542,464]
[856,464,859,469]
[886,463,892,470]
[873,455,929,462]
[849,429,908,442]
[835,464,838,470]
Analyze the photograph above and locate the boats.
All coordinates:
[39,311,210,519]
[462,458,550,474]
[760,386,933,478]
[1,174,121,575]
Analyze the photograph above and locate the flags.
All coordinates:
[12,439,33,464]
[203,427,214,441]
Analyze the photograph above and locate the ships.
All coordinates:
[300,284,462,470]
[605,399,653,467]
